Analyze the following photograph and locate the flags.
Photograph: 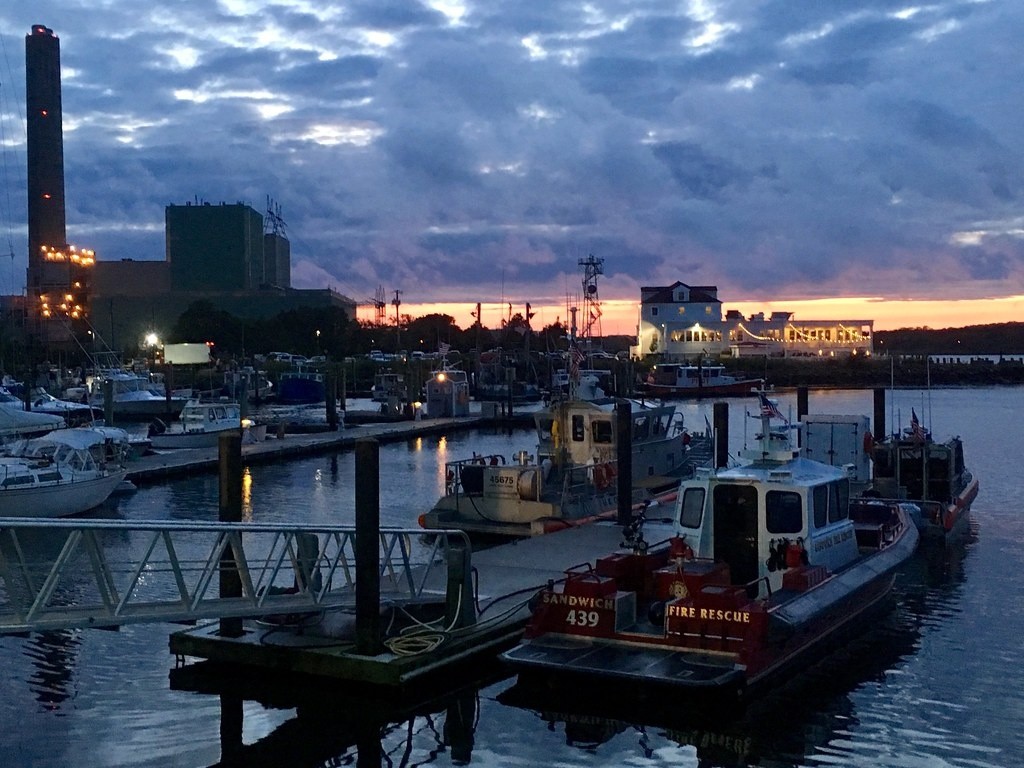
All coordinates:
[571,348,586,365]
[909,407,926,453]
[759,394,788,424]
[570,363,580,381]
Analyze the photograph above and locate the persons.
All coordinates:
[861,483,879,498]
[34,397,45,407]
[82,390,87,405]
[131,358,135,373]
[403,401,413,421]
[93,361,106,378]
[0,366,20,392]
[42,360,83,384]
[208,352,240,375]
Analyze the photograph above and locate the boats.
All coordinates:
[0,322,797,548]
[828,354,981,543]
[498,401,922,714]
